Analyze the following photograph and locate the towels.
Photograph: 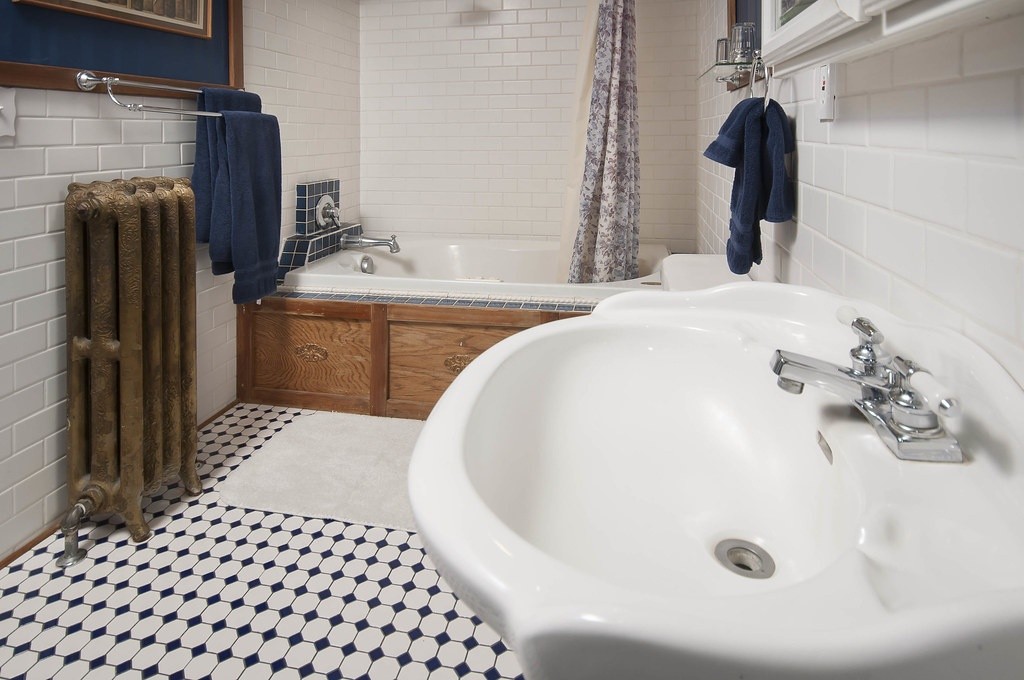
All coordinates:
[702,99,797,278]
[188,85,284,308]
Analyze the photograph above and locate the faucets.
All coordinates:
[764,316,970,470]
[339,233,401,254]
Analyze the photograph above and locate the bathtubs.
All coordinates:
[280,238,673,302]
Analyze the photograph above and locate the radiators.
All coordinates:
[63,176,203,544]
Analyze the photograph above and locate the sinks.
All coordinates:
[402,276,1024,680]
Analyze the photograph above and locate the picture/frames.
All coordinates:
[23,0,213,40]
[0,0,245,104]
[725,0,767,93]
